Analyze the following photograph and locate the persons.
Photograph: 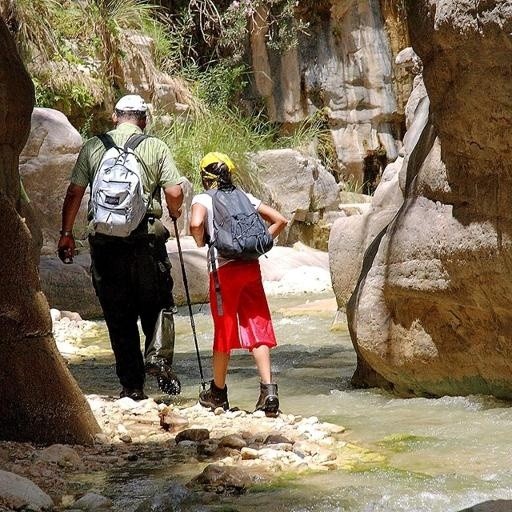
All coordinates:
[189,151,287,413]
[57,94,187,400]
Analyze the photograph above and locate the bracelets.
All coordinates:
[59,230,72,237]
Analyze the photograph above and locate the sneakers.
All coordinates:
[144,356,181,396]
[255,381,281,413]
[199,380,228,411]
[119,387,147,400]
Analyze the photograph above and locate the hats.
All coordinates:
[200,150,235,185]
[114,95,147,112]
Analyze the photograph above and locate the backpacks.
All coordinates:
[85,132,156,239]
[205,188,274,261]
